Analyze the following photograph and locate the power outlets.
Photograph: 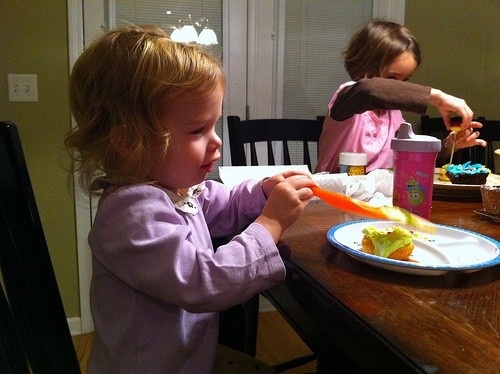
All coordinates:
[8,74,38,103]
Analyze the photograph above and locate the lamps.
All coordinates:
[169,9,219,46]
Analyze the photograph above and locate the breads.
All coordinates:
[362,236,414,261]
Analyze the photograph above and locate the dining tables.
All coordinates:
[214,186,500,374]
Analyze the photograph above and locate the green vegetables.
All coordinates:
[362,226,412,258]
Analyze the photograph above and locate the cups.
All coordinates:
[481,186,500,215]
[340,152,368,175]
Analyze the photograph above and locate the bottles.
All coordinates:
[390,121,442,222]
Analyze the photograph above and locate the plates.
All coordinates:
[432,167,500,199]
[473,210,500,221]
[326,218,500,275]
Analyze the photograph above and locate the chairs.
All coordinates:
[0,120,82,374]
[420,114,500,172]
[227,115,325,174]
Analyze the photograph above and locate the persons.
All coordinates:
[65,25,315,374]
[315,19,486,174]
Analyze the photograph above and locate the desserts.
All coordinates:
[438,161,491,185]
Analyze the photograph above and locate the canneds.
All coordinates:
[339,152,367,176]
